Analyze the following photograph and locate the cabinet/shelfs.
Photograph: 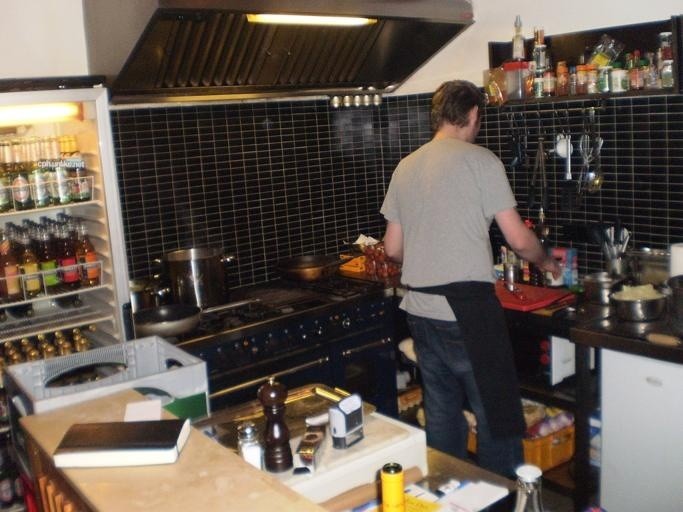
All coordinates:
[0,78,135,425]
[380,296,602,498]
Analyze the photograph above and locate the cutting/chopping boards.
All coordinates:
[493,279,569,312]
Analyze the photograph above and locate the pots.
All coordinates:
[149,247,235,308]
[131,294,265,340]
[272,241,384,284]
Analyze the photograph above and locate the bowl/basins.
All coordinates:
[606,289,671,322]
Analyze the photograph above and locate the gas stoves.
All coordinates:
[122,269,387,377]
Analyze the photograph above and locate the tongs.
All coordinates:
[543,291,580,310]
[526,135,548,211]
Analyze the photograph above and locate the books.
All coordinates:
[51,419,191,469]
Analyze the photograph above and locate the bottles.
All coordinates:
[56,295,84,308]
[237,422,266,471]
[0,232,21,303]
[523,220,536,284]
[11,138,31,211]
[6,434,25,501]
[587,64,598,94]
[5,329,65,362]
[0,212,87,244]
[17,228,41,295]
[640,60,650,83]
[52,135,71,205]
[381,463,405,512]
[70,134,90,202]
[79,339,96,383]
[530,233,545,286]
[625,53,631,70]
[63,341,77,385]
[515,464,543,512]
[29,138,49,209]
[49,136,59,206]
[0,309,7,322]
[10,303,34,317]
[0,141,10,212]
[557,66,567,95]
[630,50,644,91]
[661,60,676,89]
[569,66,577,96]
[659,32,673,60]
[37,225,59,292]
[611,62,628,95]
[0,438,13,508]
[534,78,543,98]
[542,72,554,97]
[512,28,525,62]
[503,62,534,101]
[72,323,97,341]
[80,220,100,286]
[598,66,612,95]
[534,45,546,73]
[60,223,81,289]
[576,66,588,95]
[0,357,10,426]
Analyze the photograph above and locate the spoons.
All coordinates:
[584,108,603,196]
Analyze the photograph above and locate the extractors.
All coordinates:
[82,0,475,106]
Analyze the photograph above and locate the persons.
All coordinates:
[380,81,561,481]
